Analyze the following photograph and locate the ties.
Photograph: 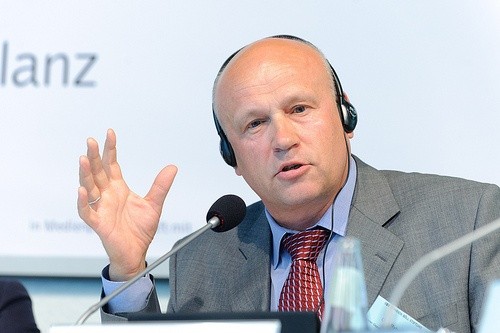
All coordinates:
[278,230,330,322]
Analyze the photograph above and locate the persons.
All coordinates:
[0,280,40,333]
[77,34,500,332]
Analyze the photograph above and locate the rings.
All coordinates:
[89,196,101,205]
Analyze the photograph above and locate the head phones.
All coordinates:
[213,35,357,167]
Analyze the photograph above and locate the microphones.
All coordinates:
[74,194,247,324]
